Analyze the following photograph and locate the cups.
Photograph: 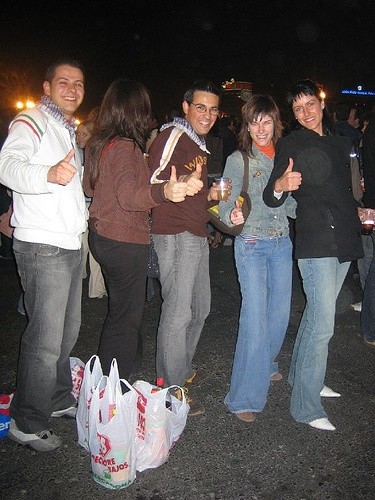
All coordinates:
[360,209,375,235]
[214,177,229,201]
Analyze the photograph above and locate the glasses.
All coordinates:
[191,102,221,116]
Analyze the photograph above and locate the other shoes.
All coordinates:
[350,301,362,312]
[308,417,335,430]
[270,373,282,381]
[364,335,375,346]
[318,386,341,397]
[235,410,255,422]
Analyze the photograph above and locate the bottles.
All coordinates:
[150,378,172,412]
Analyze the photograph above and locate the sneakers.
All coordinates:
[8,417,62,453]
[50,406,76,419]
[185,369,209,384]
[160,385,206,417]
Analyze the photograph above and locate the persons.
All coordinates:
[144,82,232,412]
[141,112,159,152]
[0,60,101,451]
[76,120,95,148]
[263,77,365,430]
[322,98,375,345]
[82,79,187,395]
[218,94,298,421]
[206,117,240,189]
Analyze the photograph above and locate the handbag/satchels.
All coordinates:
[206,151,251,236]
[65,355,189,489]
